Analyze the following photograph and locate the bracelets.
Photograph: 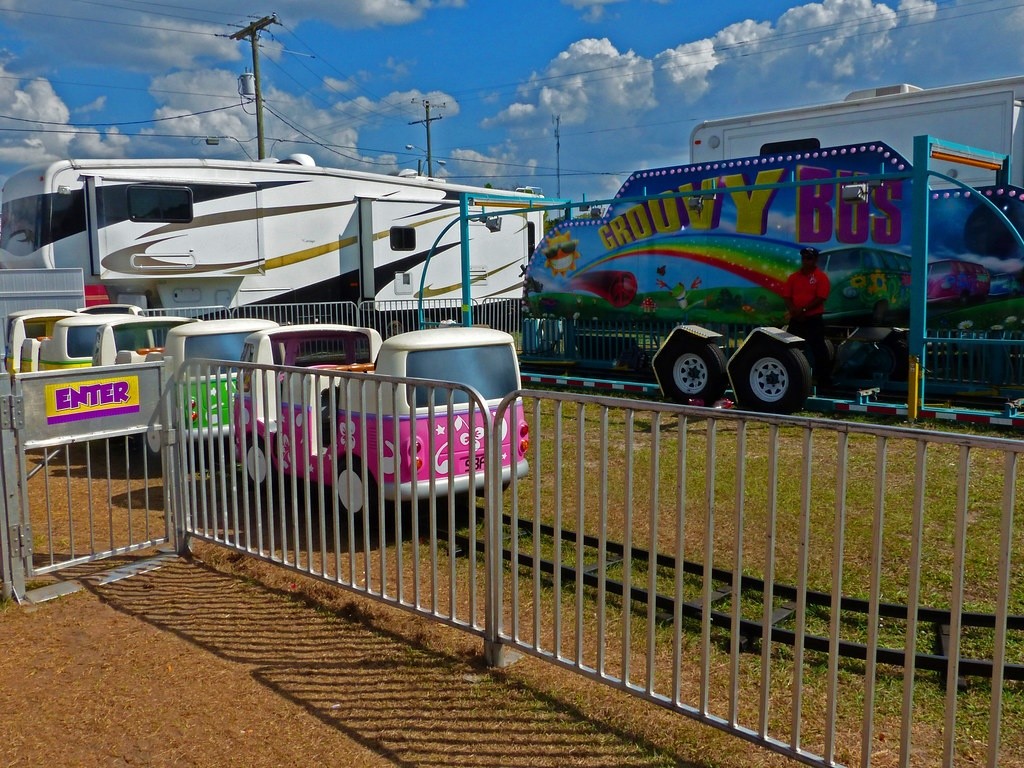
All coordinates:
[802,307,806,312]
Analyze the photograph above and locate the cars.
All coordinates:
[5,300,529,528]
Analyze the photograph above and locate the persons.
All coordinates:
[784,245,831,396]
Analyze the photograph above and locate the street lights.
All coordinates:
[416,158,446,177]
[406,143,433,179]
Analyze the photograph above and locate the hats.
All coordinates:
[799,246,819,257]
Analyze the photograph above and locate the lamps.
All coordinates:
[688,196,703,210]
[486,217,502,232]
[841,182,869,205]
[591,208,601,217]
